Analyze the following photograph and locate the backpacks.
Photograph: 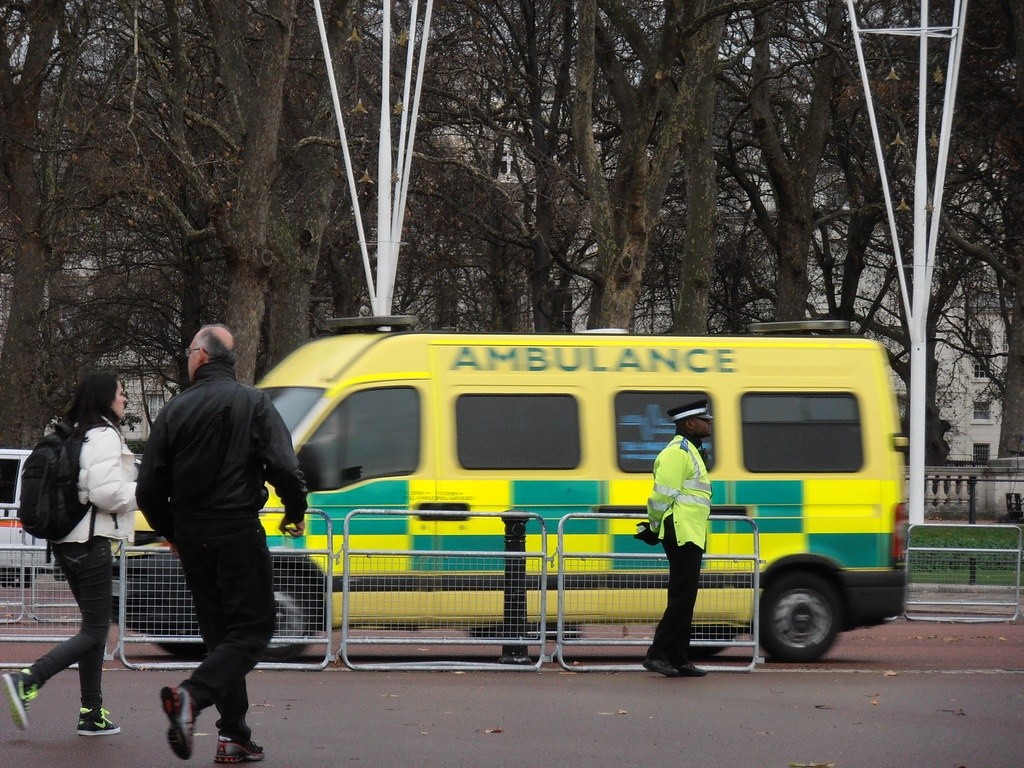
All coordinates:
[17,421,120,541]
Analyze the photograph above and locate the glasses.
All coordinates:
[185,347,209,357]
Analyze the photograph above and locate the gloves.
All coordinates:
[633,521,660,546]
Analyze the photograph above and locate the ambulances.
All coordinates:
[106,317,909,667]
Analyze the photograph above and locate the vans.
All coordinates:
[0,450,61,589]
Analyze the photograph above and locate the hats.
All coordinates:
[666,398,714,423]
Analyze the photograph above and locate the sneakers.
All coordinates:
[642,656,679,677]
[160,686,195,760]
[666,663,707,677]
[76,706,121,736]
[213,736,265,764]
[0,668,39,729]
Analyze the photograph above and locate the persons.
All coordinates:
[633,400,716,677]
[1,372,141,736]
[134,325,307,764]
